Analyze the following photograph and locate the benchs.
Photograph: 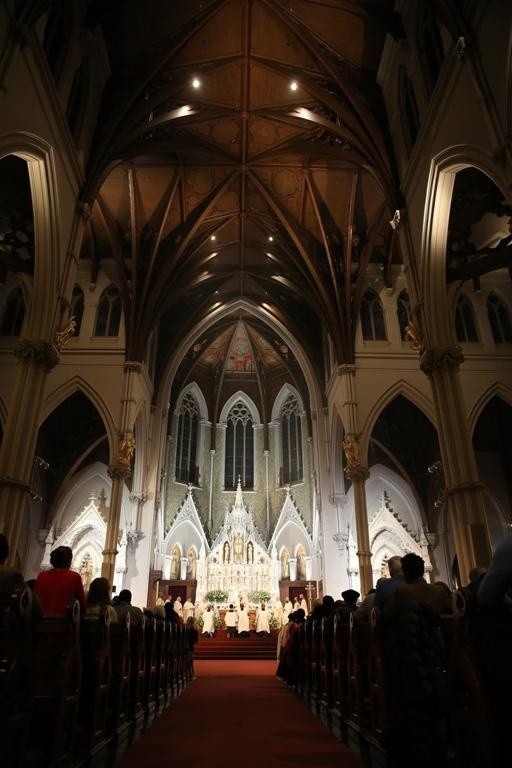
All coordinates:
[0,610,512,768]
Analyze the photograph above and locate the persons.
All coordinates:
[1,535,199,638]
[279,535,512,638]
[199,597,273,638]
